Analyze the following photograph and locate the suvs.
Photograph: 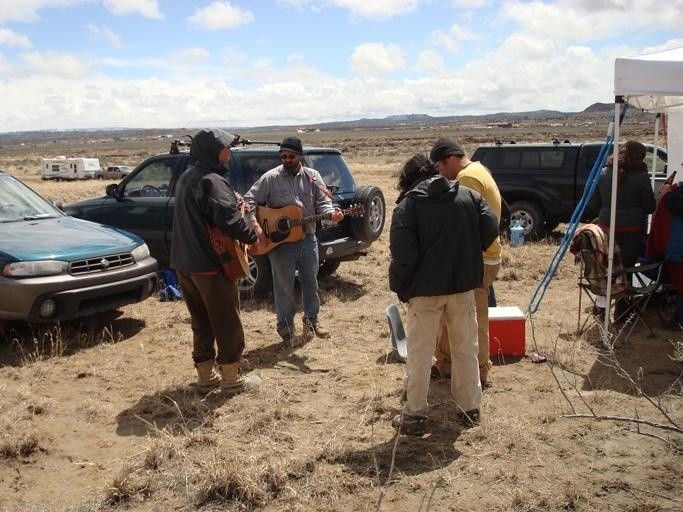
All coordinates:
[55,136,386,302]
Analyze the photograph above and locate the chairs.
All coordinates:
[578,234,665,341]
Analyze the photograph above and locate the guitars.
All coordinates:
[205,190,249,280]
[247,204,365,256]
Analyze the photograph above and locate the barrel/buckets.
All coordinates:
[510,221,524,245]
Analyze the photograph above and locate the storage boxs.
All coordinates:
[488,306,526,356]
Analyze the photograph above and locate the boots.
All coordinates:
[277,321,304,347]
[303,317,330,338]
[193,359,260,393]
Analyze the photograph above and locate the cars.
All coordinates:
[0,169,158,327]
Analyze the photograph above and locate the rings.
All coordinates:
[659,191,661,194]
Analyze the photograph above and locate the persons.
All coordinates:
[169,127,262,397]
[431,139,502,391]
[244,135,344,349]
[389,153,500,437]
[658,181,683,329]
[593,141,657,324]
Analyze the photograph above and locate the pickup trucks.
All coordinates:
[93,166,132,182]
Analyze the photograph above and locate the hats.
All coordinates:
[431,138,465,161]
[279,137,303,153]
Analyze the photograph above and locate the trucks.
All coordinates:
[39,157,99,181]
[468,141,667,245]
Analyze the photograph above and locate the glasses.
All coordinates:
[281,154,296,159]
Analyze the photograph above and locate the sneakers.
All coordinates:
[392,414,424,435]
[458,409,479,427]
